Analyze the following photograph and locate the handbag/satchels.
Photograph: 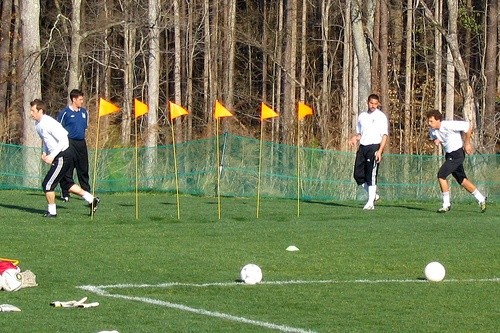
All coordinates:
[0,258,23,292]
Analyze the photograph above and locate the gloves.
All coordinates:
[0,304,21,312]
[50,301,76,307]
[73,297,99,308]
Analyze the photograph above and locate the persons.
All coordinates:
[56,89,91,200]
[428,109,487,212]
[30,98,100,217]
[348,94,389,209]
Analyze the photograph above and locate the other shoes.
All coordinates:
[63,197,69,202]
[363,205,374,210]
[374,194,380,201]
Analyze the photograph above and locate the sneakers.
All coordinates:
[437,204,451,213]
[479,196,487,213]
[42,211,57,218]
[84,197,101,216]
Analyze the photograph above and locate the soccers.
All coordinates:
[425,262,445,282]
[2,269,23,291]
[240,264,262,284]
[0,274,2,291]
